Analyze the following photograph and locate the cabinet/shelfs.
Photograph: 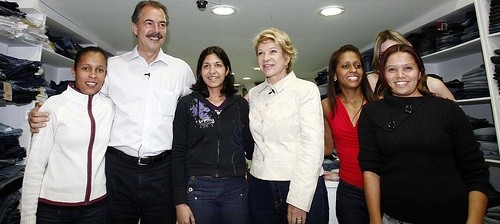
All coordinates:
[0,0,500,224]
